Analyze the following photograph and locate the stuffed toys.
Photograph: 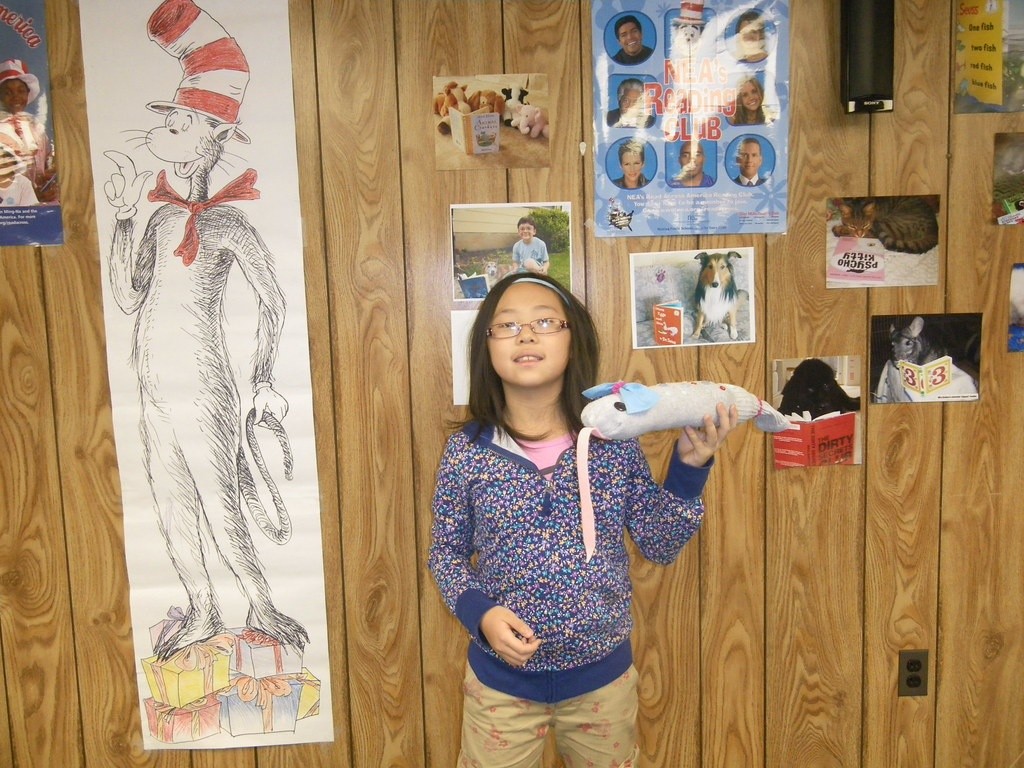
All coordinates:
[577,380,791,562]
[433,80,550,138]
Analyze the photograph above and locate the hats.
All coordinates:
[0,123,26,174]
[0,59,40,110]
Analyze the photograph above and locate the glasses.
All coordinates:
[485,318,570,339]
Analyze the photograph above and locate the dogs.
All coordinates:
[691,251,749,341]
[481,258,514,287]
[668,23,704,60]
[776,358,860,421]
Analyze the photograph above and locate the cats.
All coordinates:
[832,195,939,255]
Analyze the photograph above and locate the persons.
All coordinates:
[614,140,649,189]
[733,137,766,187]
[732,13,769,63]
[502,217,550,278]
[668,140,716,188]
[728,75,772,125]
[0,60,58,206]
[611,15,652,66]
[608,78,656,128]
[427,271,738,768]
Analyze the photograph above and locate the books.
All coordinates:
[826,236,885,284]
[899,355,952,397]
[457,272,491,298]
[653,300,684,346]
[448,106,500,155]
[774,411,856,470]
[997,193,1024,225]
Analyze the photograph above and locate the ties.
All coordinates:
[747,181,753,186]
